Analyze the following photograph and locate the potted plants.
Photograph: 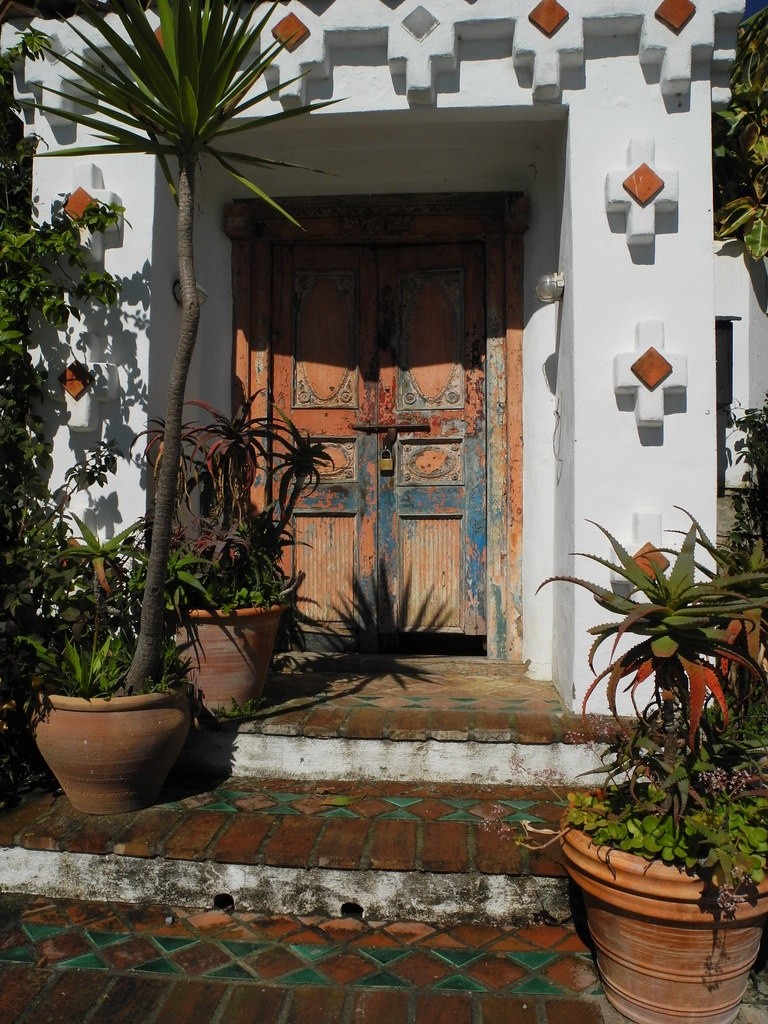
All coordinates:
[0,0,768,1024]
